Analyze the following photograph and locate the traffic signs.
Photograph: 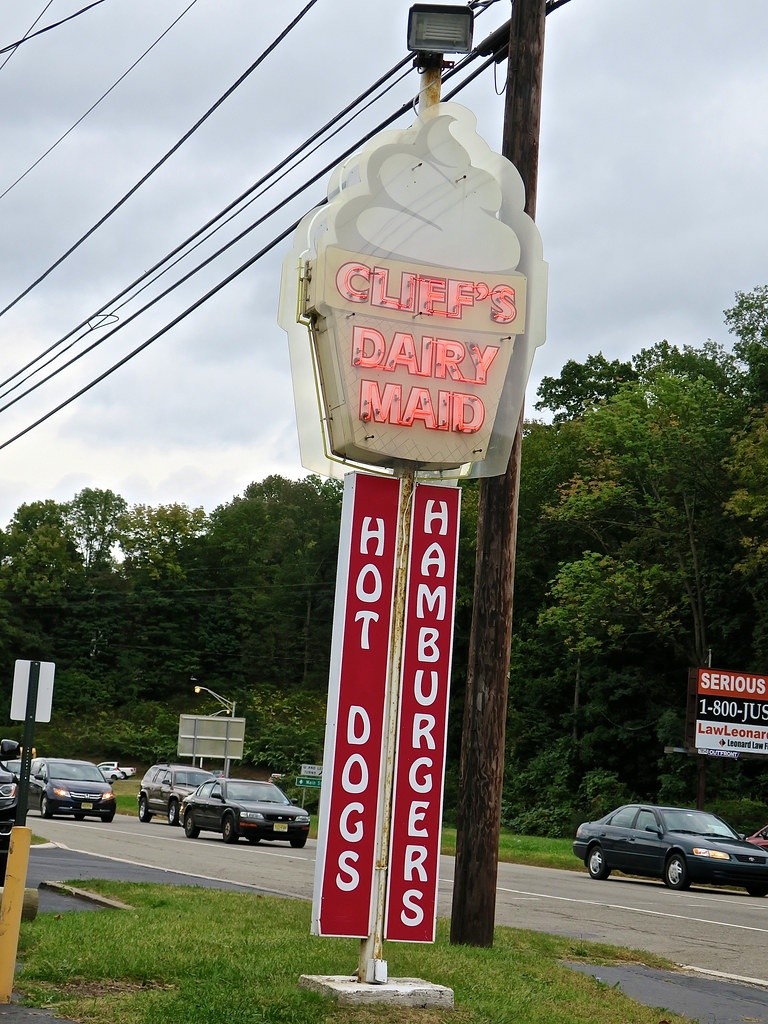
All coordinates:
[296,776,321,788]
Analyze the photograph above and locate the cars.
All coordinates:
[0,739,20,887]
[1,760,21,782]
[26,758,117,823]
[745,825,768,849]
[98,766,123,780]
[179,778,310,849]
[572,804,768,896]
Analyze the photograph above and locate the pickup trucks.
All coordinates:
[96,762,137,780]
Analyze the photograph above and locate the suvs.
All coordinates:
[137,762,220,827]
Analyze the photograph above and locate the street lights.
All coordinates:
[199,710,229,769]
[195,685,236,779]
[277,3,552,981]
